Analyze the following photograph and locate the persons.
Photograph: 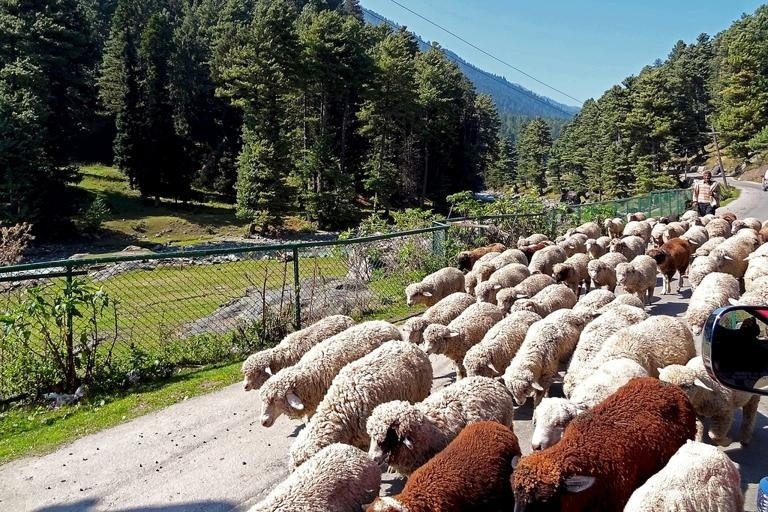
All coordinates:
[693,170,720,216]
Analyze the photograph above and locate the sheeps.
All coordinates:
[365,375,515,474]
[246,442,381,512]
[406,267,466,308]
[657,353,761,447]
[402,291,476,345]
[678,271,740,338]
[475,248,529,286]
[559,304,650,398]
[517,210,768,306]
[620,440,750,511]
[286,341,430,476]
[362,421,524,512]
[577,312,697,379]
[512,284,581,319]
[502,305,581,409]
[466,251,500,294]
[459,242,507,272]
[531,358,649,452]
[259,316,402,429]
[462,309,542,379]
[726,275,767,309]
[495,272,557,312]
[423,299,505,380]
[510,373,692,512]
[240,312,356,393]
